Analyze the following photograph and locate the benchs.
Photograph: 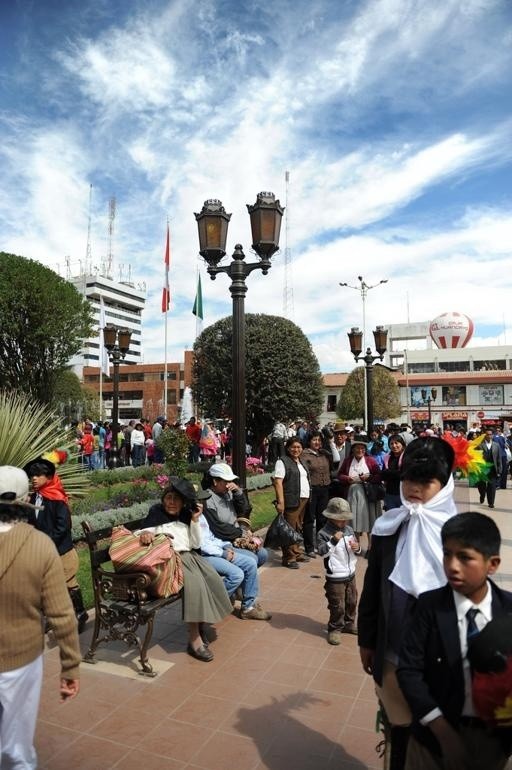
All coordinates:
[79,516,224,678]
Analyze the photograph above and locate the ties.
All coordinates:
[466,608,480,639]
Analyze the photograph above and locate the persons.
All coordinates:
[1,464,82,770]
[20,413,238,470]
[355,433,460,770]
[317,497,362,646]
[392,510,512,770]
[244,413,510,569]
[138,464,272,664]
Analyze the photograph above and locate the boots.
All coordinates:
[69,589,89,634]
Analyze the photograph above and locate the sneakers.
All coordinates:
[282,561,299,569]
[328,632,341,645]
[240,603,272,620]
[296,555,310,562]
[342,627,359,635]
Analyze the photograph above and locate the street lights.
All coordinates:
[100,327,136,482]
[189,191,287,543]
[349,324,395,468]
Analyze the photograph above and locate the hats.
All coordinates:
[399,436,454,484]
[172,477,196,499]
[157,416,167,422]
[332,422,349,433]
[321,496,353,521]
[351,439,369,445]
[209,463,241,481]
[1,465,30,505]
[30,460,55,475]
[190,480,212,500]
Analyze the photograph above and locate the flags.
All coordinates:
[161,222,170,312]
[191,271,203,333]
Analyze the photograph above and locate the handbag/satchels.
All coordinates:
[363,481,386,502]
[233,517,263,553]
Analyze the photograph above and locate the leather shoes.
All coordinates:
[187,643,214,662]
[307,552,316,558]
[200,630,210,647]
